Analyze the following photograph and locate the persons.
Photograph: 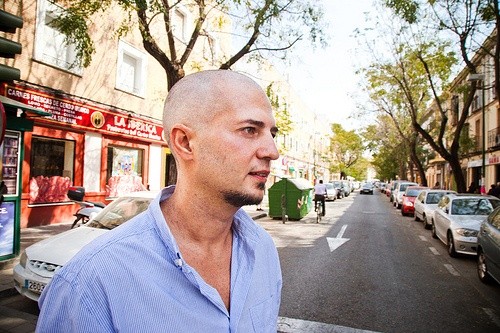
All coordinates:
[35,69,283,333]
[315,179,327,216]
[433,182,440,189]
[468,182,477,194]
[486,182,500,199]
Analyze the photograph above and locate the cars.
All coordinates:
[400,186,432,217]
[359,183,374,195]
[476,207,500,286]
[430,193,500,259]
[374,180,419,209]
[322,180,361,201]
[11,189,158,305]
[414,190,458,230]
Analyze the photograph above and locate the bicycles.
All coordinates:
[314,195,330,223]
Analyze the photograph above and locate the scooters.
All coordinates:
[67,186,106,231]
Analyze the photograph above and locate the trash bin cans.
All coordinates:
[268,179,314,221]
